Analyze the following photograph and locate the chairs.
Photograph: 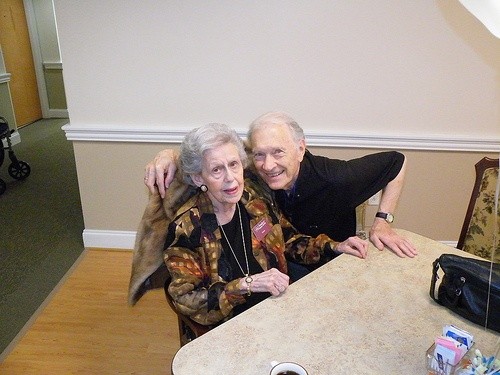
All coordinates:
[456,158,500,266]
[152,173,207,346]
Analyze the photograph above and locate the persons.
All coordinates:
[144,112,416,286]
[161,123,368,348]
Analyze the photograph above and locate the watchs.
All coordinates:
[375,211,394,224]
[245,276,253,295]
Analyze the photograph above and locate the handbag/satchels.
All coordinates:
[428,252,500,333]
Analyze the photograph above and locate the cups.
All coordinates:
[270,360,308,375]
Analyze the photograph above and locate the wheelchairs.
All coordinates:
[0,114,30,196]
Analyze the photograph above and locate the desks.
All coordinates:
[170,227,500,374]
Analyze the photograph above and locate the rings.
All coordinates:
[144,177,148,180]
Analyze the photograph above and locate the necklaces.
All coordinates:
[217,203,250,276]
